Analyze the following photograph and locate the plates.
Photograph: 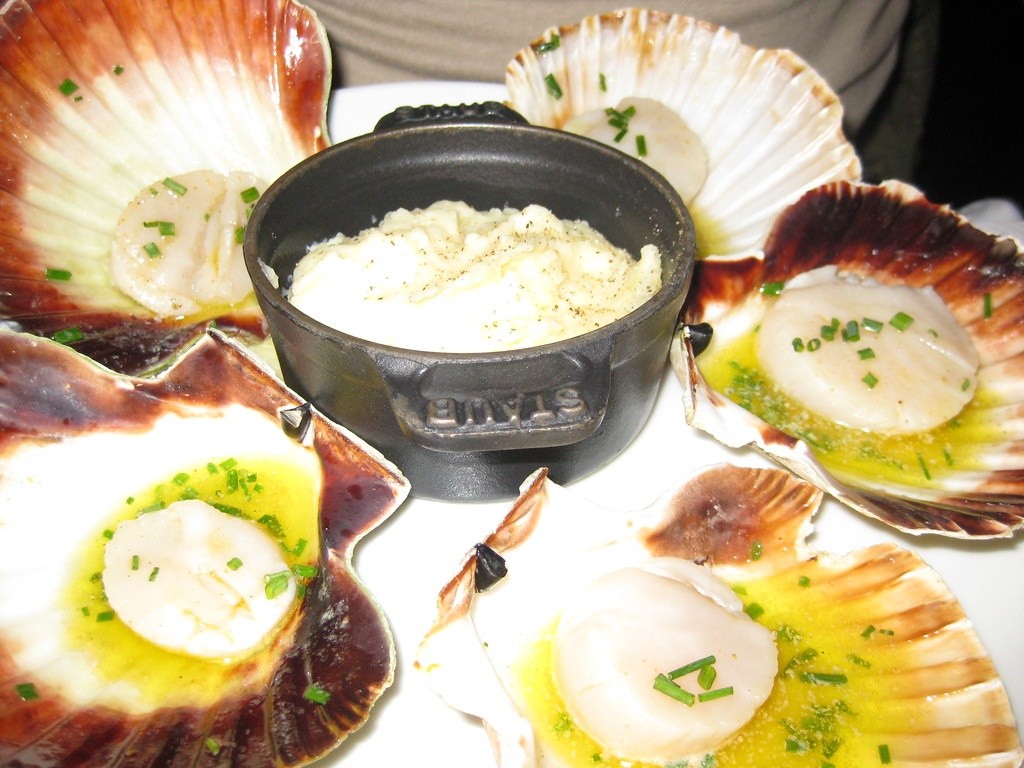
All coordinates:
[0,78,1024,768]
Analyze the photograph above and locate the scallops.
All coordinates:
[1,0,1024,767]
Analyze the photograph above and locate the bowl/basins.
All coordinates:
[244,100,698,503]
